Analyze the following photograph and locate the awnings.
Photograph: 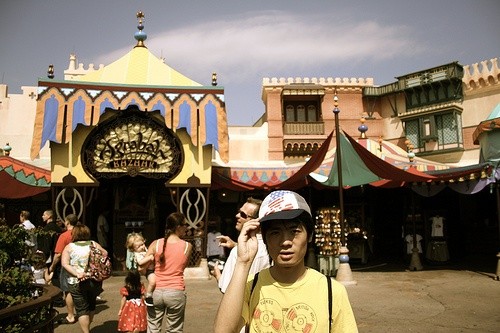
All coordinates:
[0,155,51,199]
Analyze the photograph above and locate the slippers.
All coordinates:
[57,317,74,324]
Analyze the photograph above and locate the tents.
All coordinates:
[212,89,500,246]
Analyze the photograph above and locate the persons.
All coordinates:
[117,269,147,333]
[214,190,359,333]
[209,196,274,333]
[18,209,109,333]
[137,212,194,333]
[125,231,156,307]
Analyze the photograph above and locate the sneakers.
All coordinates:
[144,297,153,306]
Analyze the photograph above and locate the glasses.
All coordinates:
[237,209,257,220]
[182,223,186,227]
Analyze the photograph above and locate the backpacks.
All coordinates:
[77,241,112,281]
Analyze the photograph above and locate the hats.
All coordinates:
[258,190,313,223]
